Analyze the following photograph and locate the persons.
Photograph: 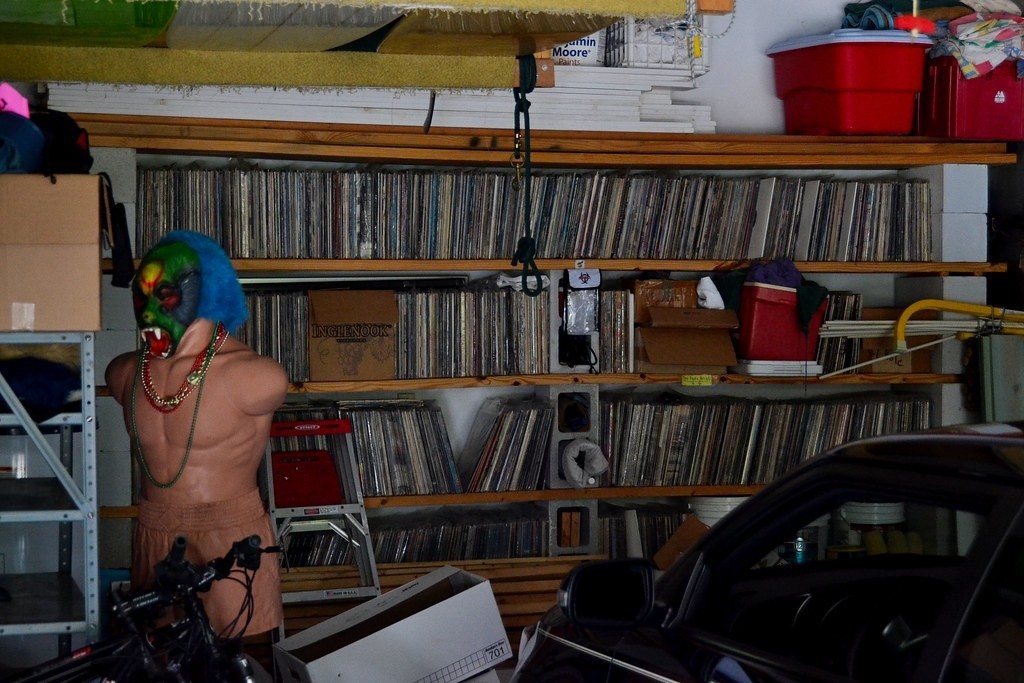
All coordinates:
[105,230,290,639]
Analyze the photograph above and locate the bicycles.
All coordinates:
[1,532,287,683]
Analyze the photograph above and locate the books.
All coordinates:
[133,168,932,565]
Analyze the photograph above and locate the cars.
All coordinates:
[505,416,1024,683]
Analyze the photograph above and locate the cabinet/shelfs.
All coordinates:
[0,331,100,656]
[135,140,942,597]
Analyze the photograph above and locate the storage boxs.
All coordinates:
[271,564,513,683]
[765,28,931,139]
[914,55,1024,142]
[859,307,936,374]
[737,281,829,361]
[634,279,697,323]
[308,289,399,381]
[0,172,114,331]
